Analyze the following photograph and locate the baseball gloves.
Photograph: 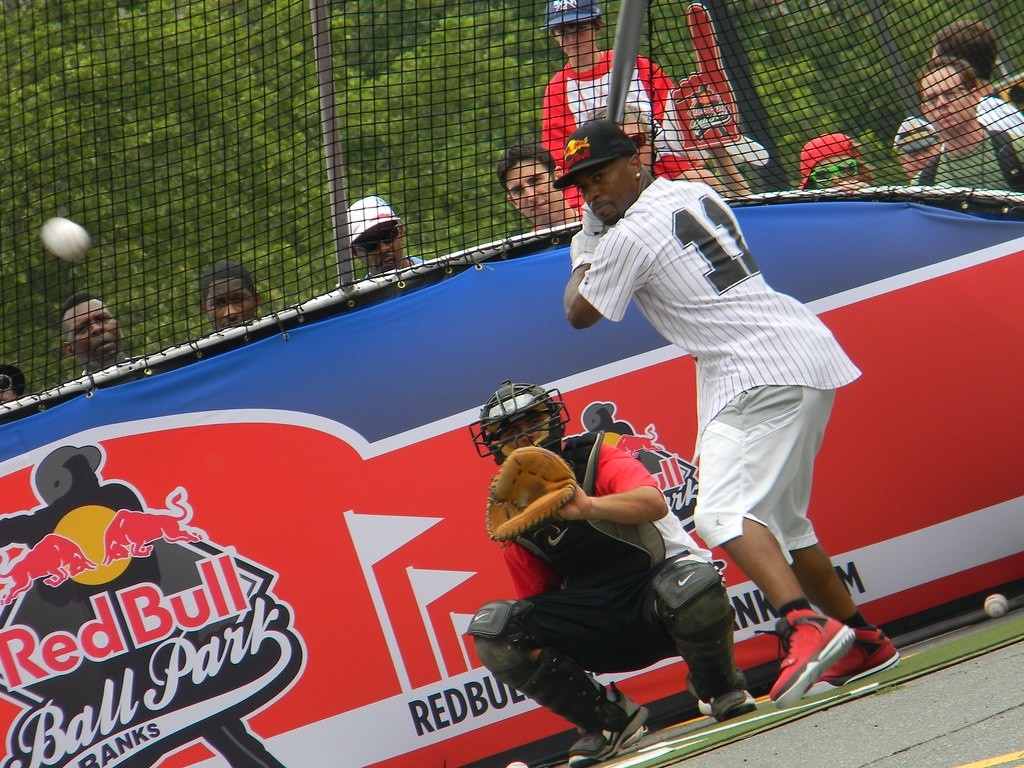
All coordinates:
[485,447,577,544]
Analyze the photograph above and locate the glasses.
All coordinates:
[920,84,972,112]
[0,374,12,392]
[357,223,397,253]
[809,158,859,187]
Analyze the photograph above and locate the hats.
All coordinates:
[892,119,941,157]
[0,364,25,396]
[554,119,640,188]
[799,130,857,190]
[347,196,400,247]
[540,1,602,31]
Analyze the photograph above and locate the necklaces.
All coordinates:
[944,131,985,187]
[567,51,603,114]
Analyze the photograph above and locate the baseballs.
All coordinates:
[43,217,89,261]
[983,593,1008,619]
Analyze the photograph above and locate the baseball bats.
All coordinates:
[606,0,647,125]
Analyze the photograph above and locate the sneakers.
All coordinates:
[573,701,650,767]
[699,690,756,720]
[802,622,901,699]
[755,607,857,710]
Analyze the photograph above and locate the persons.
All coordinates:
[0,362,27,407]
[197,262,260,337]
[895,22,1024,193]
[347,195,428,276]
[543,0,728,226]
[467,380,760,768]
[557,123,898,705]
[61,292,128,376]
[698,113,875,191]
[496,147,583,232]
